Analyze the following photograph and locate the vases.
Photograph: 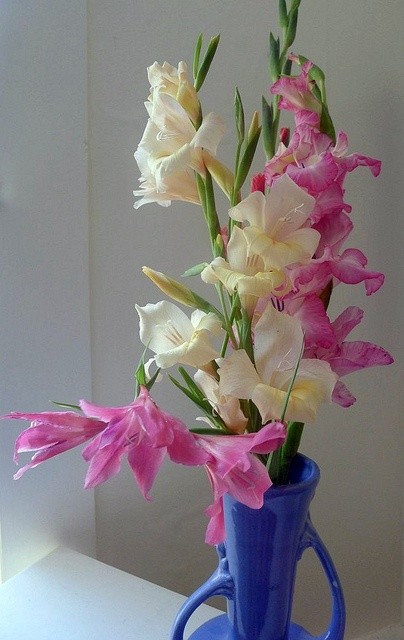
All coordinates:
[170,451,345,640]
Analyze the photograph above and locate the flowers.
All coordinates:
[0,0,394,547]
